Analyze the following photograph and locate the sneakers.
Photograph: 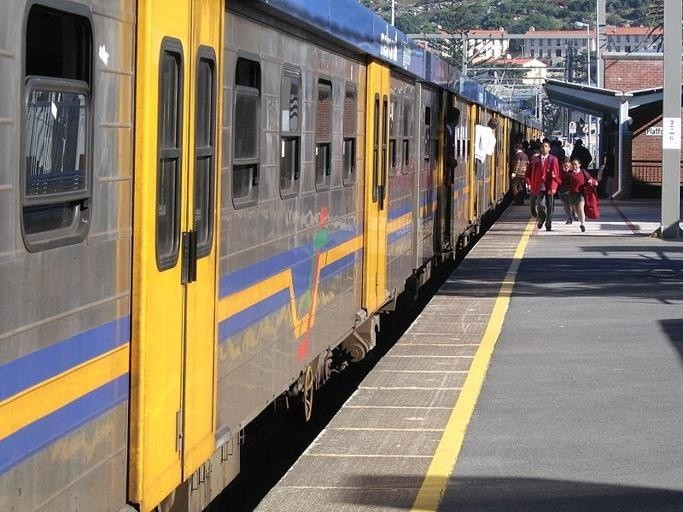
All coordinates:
[565,219,571,224]
[579,224,585,232]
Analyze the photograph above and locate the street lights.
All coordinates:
[574,21,592,150]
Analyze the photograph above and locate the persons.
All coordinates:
[568,139,592,173]
[522,140,560,232]
[473,117,500,196]
[548,157,599,233]
[508,136,565,207]
[440,107,461,253]
[553,156,579,225]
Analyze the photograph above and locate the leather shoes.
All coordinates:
[545,226,550,231]
[537,220,544,228]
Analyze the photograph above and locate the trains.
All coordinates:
[1,0,545,512]
